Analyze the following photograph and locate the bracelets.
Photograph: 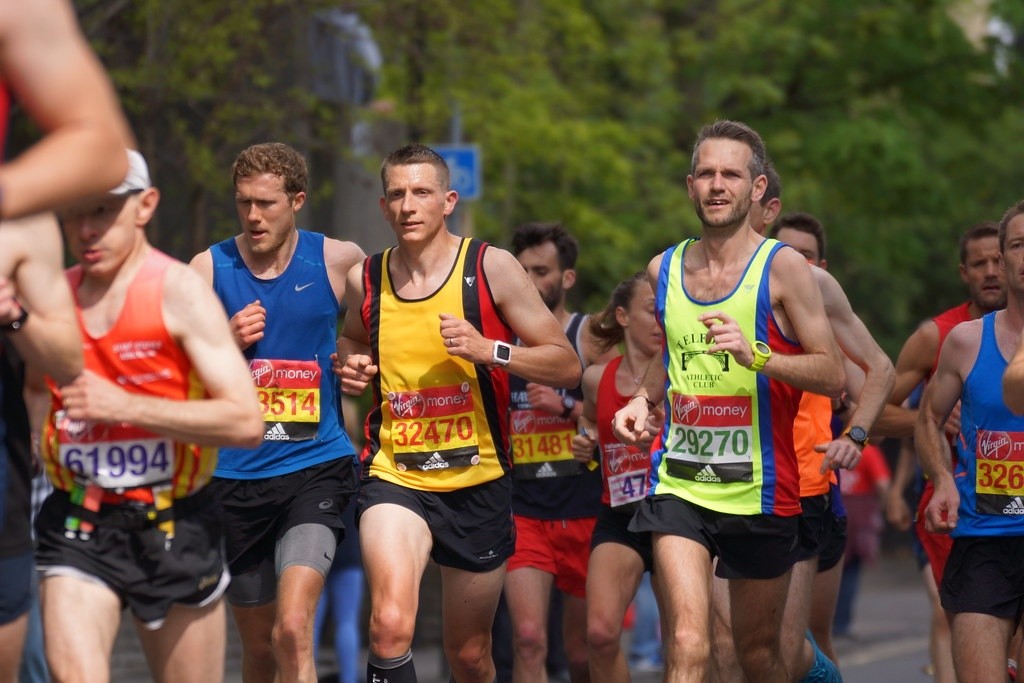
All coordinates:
[3,297,27,332]
[627,395,654,410]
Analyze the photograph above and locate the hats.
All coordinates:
[106,148,150,195]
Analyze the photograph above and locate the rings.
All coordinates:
[450,338,454,346]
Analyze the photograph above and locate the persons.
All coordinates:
[190,141,366,682]
[610,122,844,683]
[574,269,666,683]
[870,227,1007,616]
[748,166,897,683]
[503,221,603,683]
[918,201,1023,683]
[0,0,140,222]
[336,144,583,683]
[25,152,265,682]
[1,212,84,682]
[770,214,956,683]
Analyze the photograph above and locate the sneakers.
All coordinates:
[805,627,843,683]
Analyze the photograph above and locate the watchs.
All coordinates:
[558,396,576,419]
[842,426,869,449]
[493,340,512,366]
[750,339,771,372]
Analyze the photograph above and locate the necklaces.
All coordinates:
[626,369,642,386]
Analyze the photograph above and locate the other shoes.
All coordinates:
[1007,658,1018,681]
